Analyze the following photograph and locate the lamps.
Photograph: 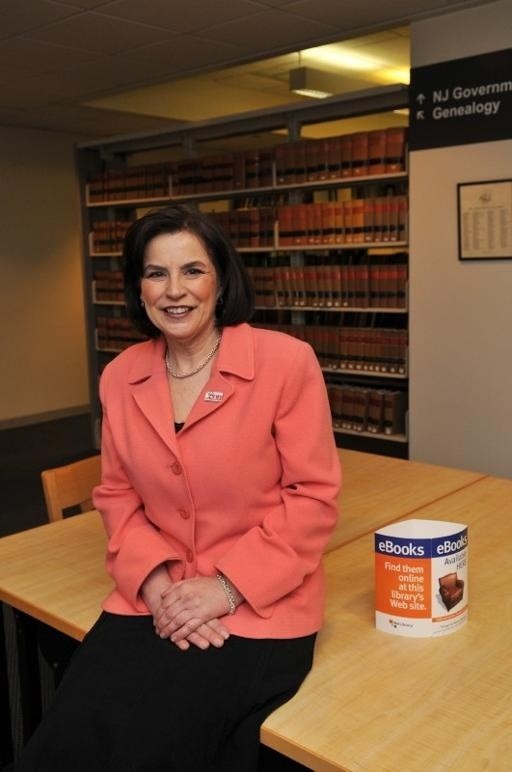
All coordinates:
[290,47,410,115]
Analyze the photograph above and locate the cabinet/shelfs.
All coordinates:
[84,162,408,444]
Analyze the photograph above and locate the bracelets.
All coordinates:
[216,574,238,614]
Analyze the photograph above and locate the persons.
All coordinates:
[91,129,407,203]
[11,205,344,771]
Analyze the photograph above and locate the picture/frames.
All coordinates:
[457,178,512,260]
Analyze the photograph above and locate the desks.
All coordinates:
[262,473,510,771]
[2,448,485,772]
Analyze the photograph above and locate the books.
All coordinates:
[94,316,407,375]
[326,380,405,435]
[91,179,410,254]
[93,263,408,307]
